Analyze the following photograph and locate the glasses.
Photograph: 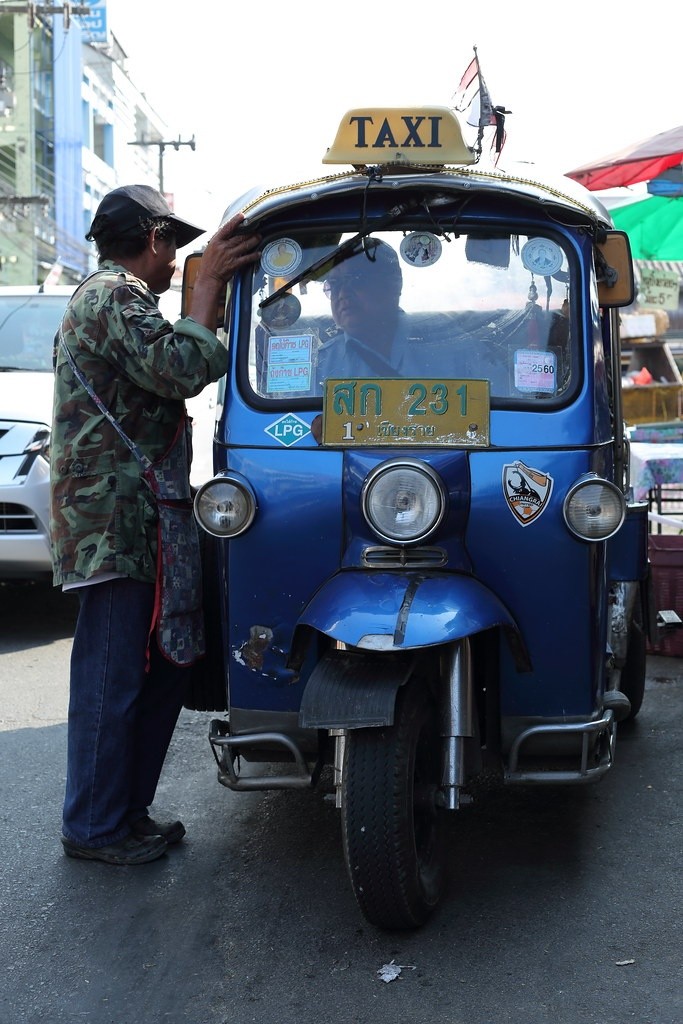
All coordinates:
[322,272,391,301]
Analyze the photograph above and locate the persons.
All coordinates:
[318,236,479,398]
[411,242,429,261]
[535,249,551,264]
[50,184,263,865]
[273,244,294,266]
[270,298,292,327]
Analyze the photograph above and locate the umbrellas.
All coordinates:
[562,125,683,261]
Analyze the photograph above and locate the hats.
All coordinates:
[91,184,206,249]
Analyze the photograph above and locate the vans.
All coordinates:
[0,284,218,624]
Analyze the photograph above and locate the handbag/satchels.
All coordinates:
[147,468,214,668]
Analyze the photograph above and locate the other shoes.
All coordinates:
[62,830,168,864]
[131,814,186,844]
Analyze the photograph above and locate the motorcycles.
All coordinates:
[180,108,655,935]
